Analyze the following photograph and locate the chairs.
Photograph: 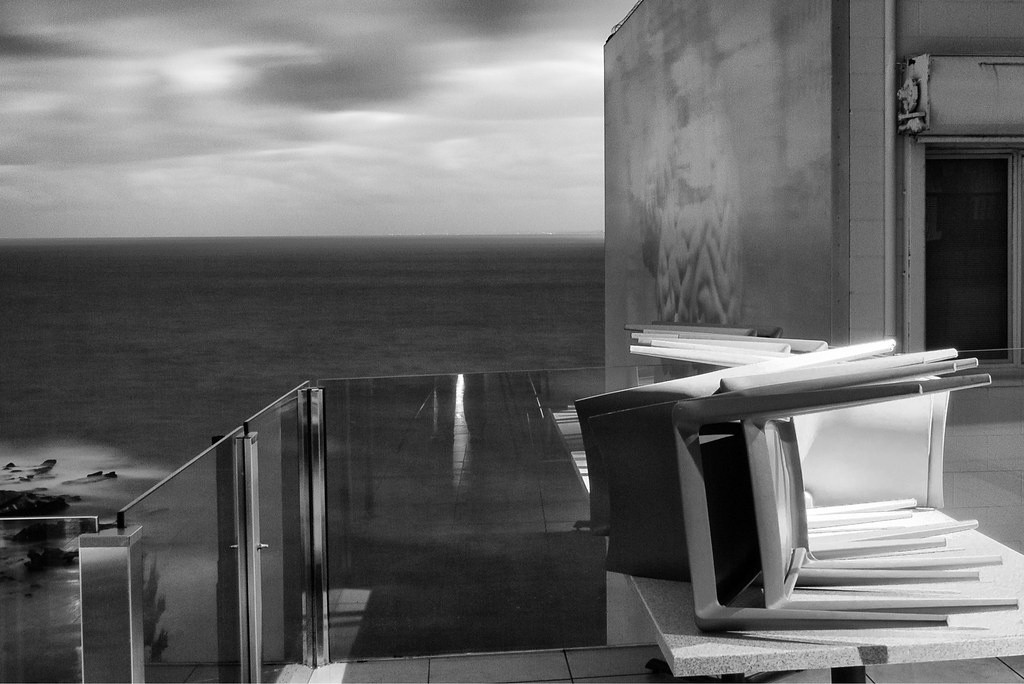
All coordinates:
[574,320,1018,636]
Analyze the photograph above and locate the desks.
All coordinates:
[567,444,1024,684]
